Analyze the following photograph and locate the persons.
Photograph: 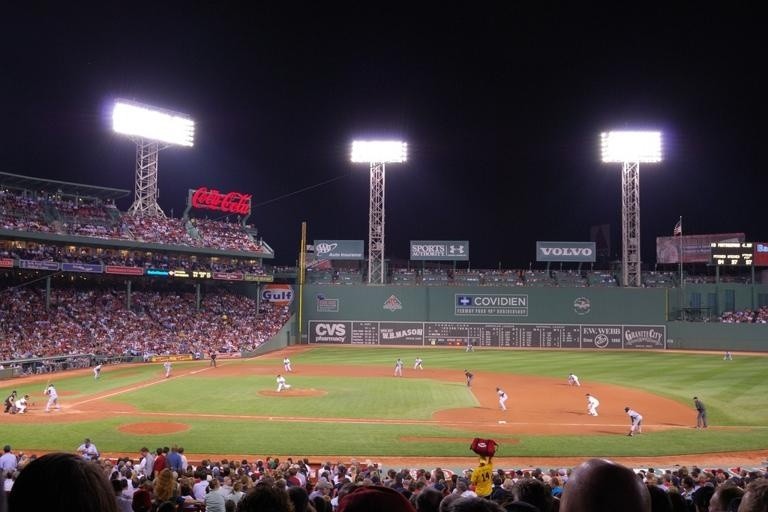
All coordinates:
[723,351,733,360]
[3,391,16,413]
[0,187,289,377]
[464,368,473,387]
[14,395,30,414]
[495,387,508,411]
[465,343,474,353]
[44,383,61,412]
[299,264,681,290]
[623,407,641,437]
[412,357,423,371]
[1,438,766,511]
[682,267,766,326]
[276,375,291,392]
[568,373,579,387]
[393,358,404,376]
[692,397,706,428]
[92,362,103,381]
[584,394,599,416]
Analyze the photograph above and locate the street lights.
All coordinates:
[107,97,195,222]
[596,129,666,291]
[347,138,411,285]
